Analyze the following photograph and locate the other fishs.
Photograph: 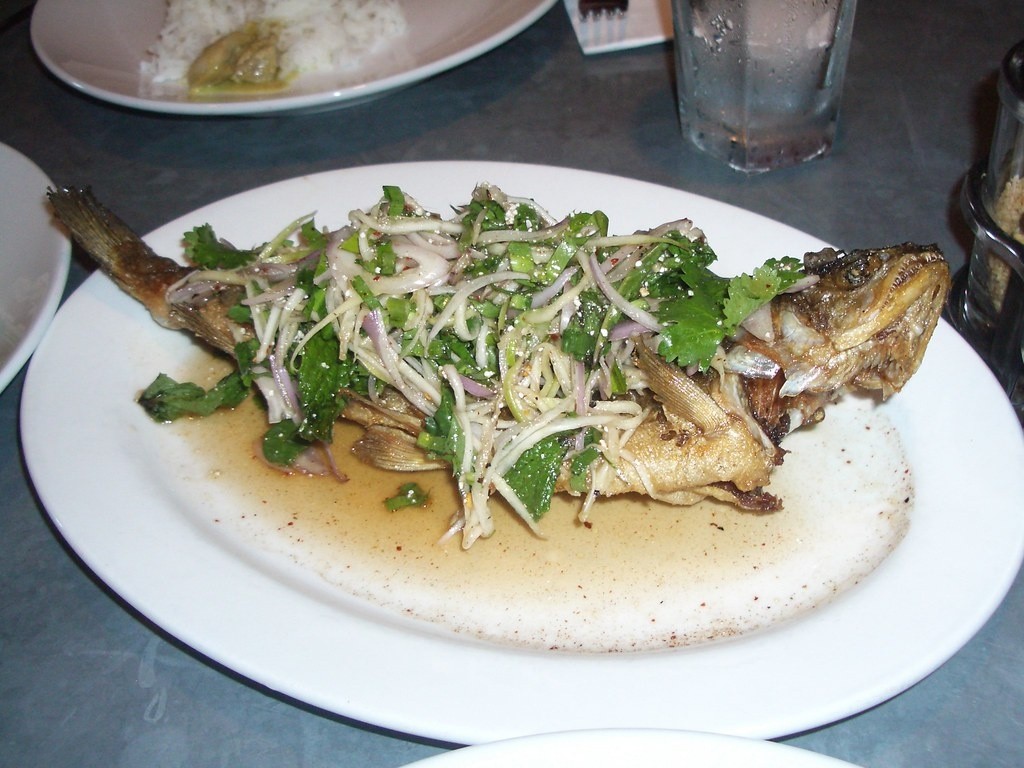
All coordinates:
[46,183,951,517]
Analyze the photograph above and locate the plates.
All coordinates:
[400,728,866,768]
[0,143,75,396]
[31,0,559,116]
[21,160,1024,743]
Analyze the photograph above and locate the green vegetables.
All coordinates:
[137,185,807,518]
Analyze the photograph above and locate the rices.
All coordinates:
[136,0,408,93]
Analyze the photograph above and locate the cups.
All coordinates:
[670,0,857,174]
[960,40,1024,407]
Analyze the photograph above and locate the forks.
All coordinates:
[578,0,629,23]
